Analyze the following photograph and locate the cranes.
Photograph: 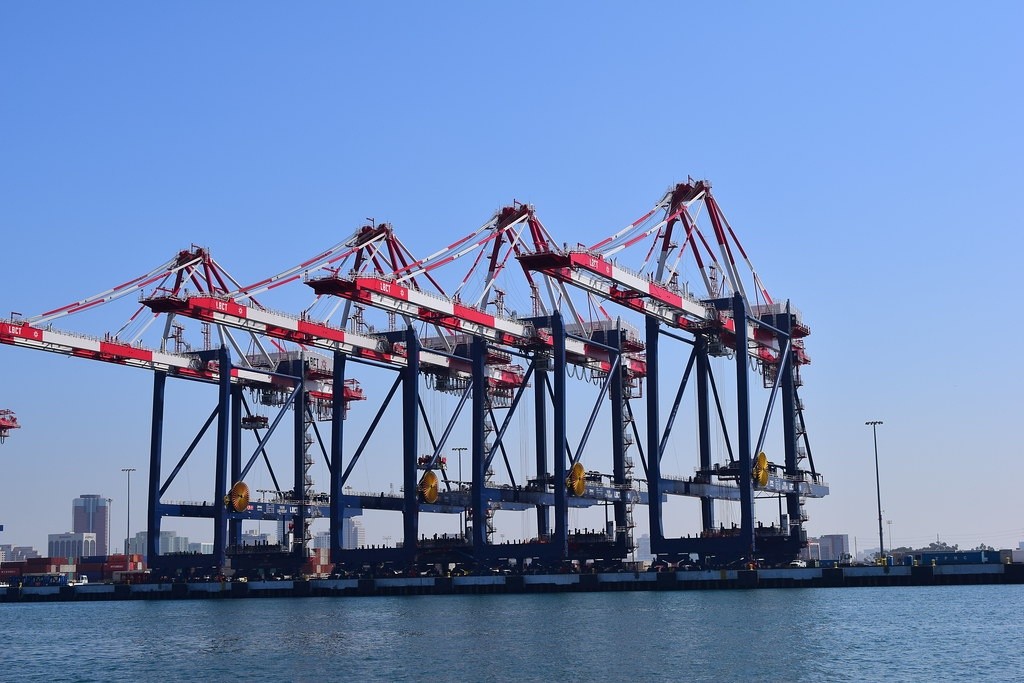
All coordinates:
[0,176,814,398]
